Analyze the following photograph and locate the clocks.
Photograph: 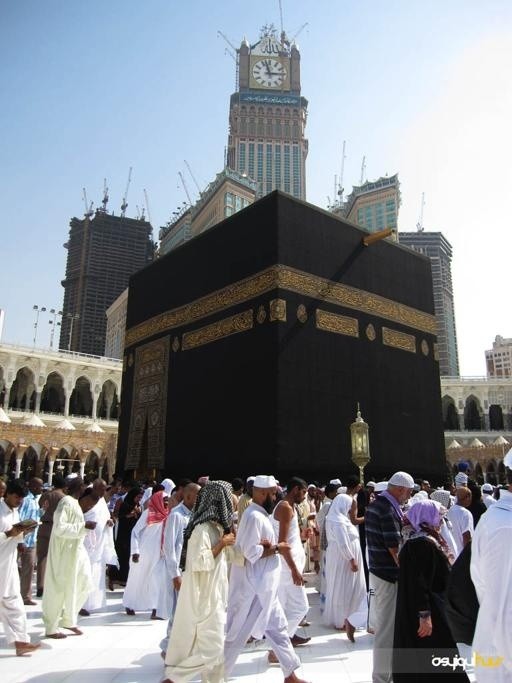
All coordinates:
[248,58,286,86]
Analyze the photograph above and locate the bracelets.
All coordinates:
[417,611,432,618]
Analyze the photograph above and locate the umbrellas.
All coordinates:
[0,407,106,433]
[446,434,510,449]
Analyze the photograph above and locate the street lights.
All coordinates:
[65,312,80,349]
[31,304,46,346]
[350,400,371,486]
[47,308,63,348]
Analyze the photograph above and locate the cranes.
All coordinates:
[82,186,94,220]
[324,137,368,207]
[100,177,109,213]
[135,204,145,221]
[120,166,133,217]
[416,190,428,232]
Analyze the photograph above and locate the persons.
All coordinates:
[154,480,237,682]
[0,456,512,650]
[159,482,203,660]
[390,500,472,683]
[0,479,43,655]
[363,471,415,682]
[455,446,512,682]
[225,475,314,683]
[266,478,311,663]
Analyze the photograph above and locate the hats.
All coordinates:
[247,476,255,483]
[374,481,389,492]
[483,484,493,491]
[330,478,342,485]
[366,481,376,487]
[388,471,415,488]
[253,475,277,488]
[307,484,316,491]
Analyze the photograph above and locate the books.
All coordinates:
[12,519,42,531]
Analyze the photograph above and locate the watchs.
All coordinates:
[274,545,280,555]
